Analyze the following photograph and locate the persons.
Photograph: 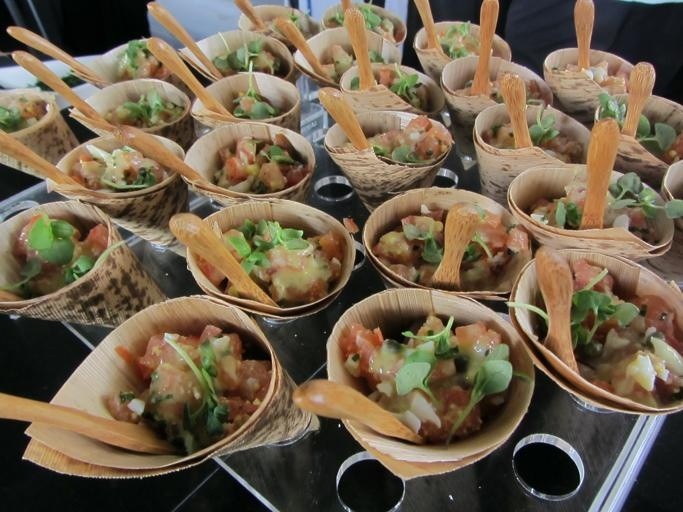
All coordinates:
[394,3,682,107]
[0,0,158,69]
